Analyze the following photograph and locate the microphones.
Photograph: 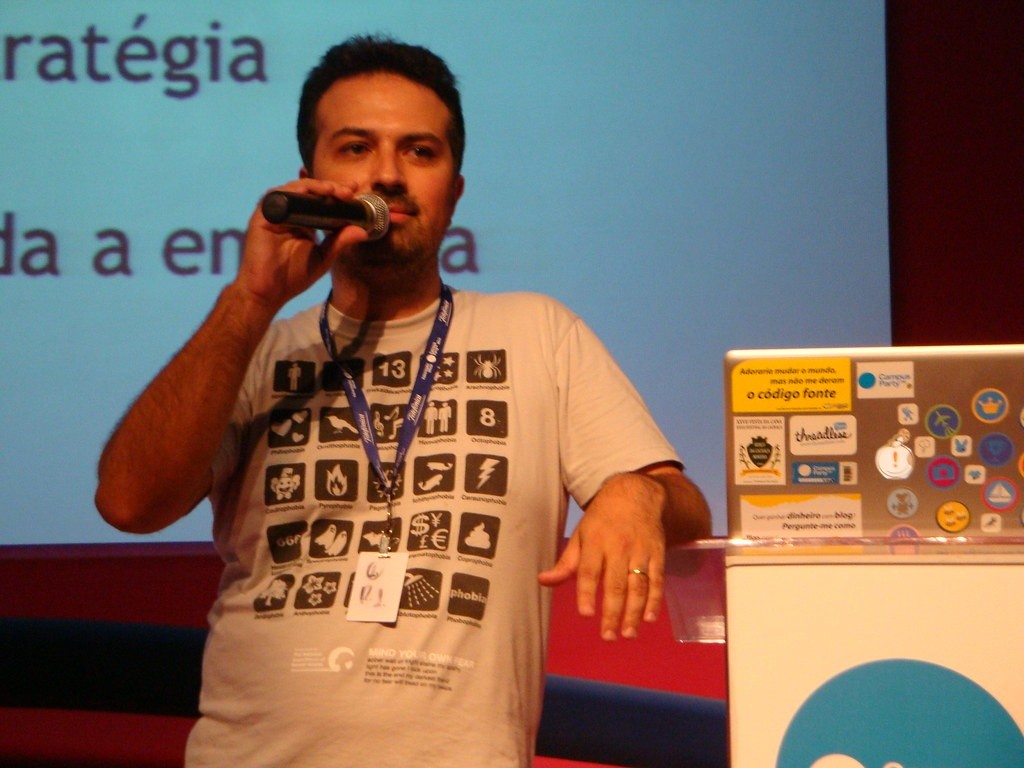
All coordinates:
[262,191,390,239]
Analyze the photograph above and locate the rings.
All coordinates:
[628,569,648,576]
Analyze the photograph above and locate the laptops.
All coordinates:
[724,344,1024,539]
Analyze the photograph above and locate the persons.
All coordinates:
[92,37,712,768]
